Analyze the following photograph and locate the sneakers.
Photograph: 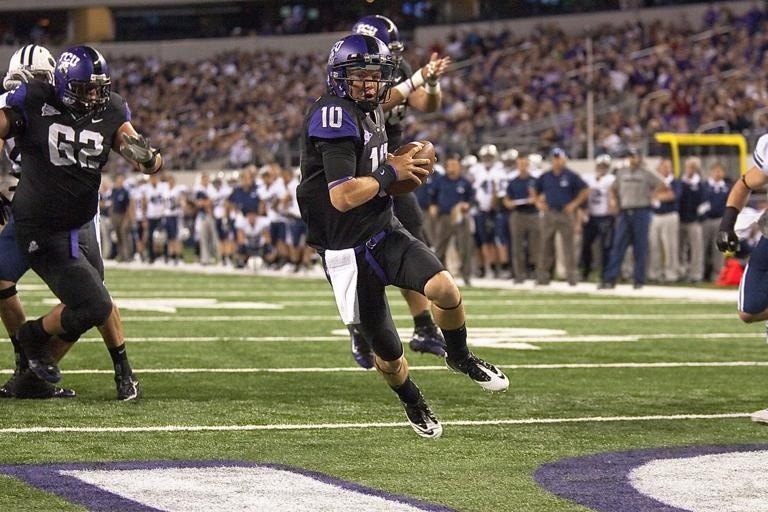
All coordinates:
[440,348,510,396]
[14,319,62,383]
[113,374,141,402]
[595,281,615,290]
[748,405,768,426]
[406,327,446,359]
[345,325,376,370]
[395,379,443,439]
[632,281,643,290]
[0,364,76,399]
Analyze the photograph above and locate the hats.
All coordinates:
[626,142,640,155]
[462,143,564,171]
[596,155,613,166]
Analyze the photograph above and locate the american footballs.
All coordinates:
[387,141,435,195]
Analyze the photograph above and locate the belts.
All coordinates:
[69,226,81,260]
[353,230,390,287]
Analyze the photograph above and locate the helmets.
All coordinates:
[326,34,395,100]
[53,46,111,102]
[351,15,406,57]
[3,44,58,91]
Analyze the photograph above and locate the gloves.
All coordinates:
[716,206,742,252]
[117,132,157,169]
